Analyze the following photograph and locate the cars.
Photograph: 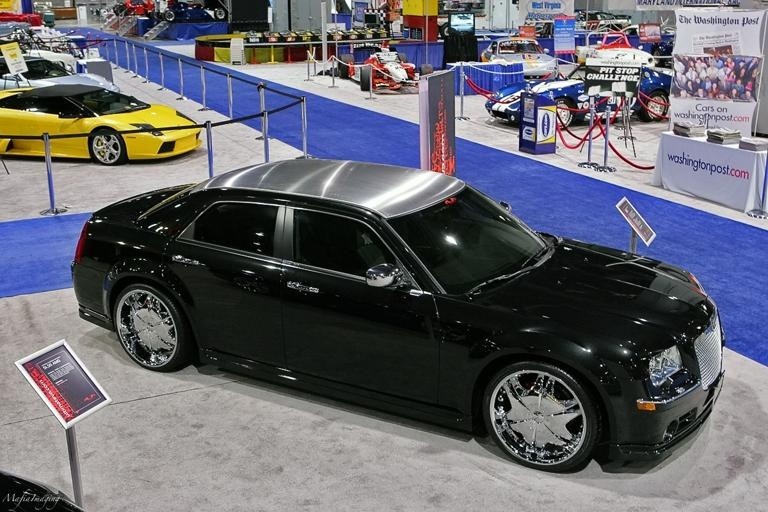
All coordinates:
[69,154,727,479]
[0,467,92,511]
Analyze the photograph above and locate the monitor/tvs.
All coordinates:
[364,12,379,24]
[447,11,475,34]
[351,0,369,25]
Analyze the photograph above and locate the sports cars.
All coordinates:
[482,36,558,80]
[489,62,676,133]
[110,0,229,23]
[0,9,209,166]
[576,32,655,69]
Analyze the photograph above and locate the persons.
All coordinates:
[672,54,759,102]
